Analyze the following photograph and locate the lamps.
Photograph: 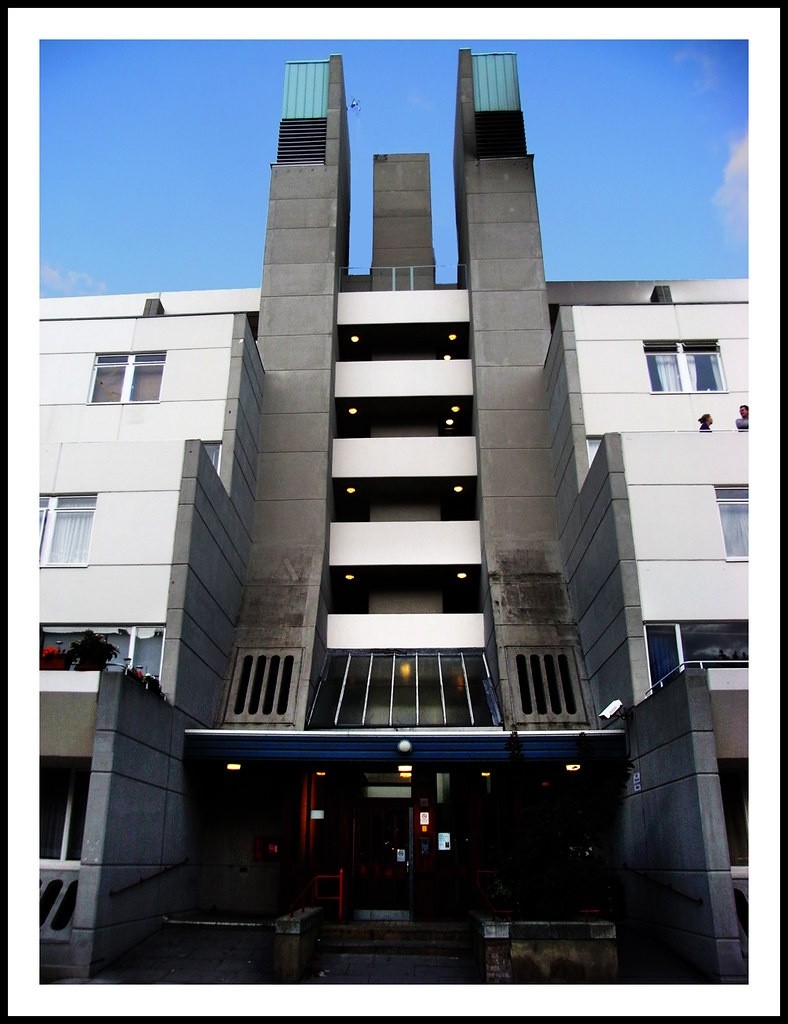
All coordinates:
[397,739,413,754]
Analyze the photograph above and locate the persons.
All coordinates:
[698,414,713,432]
[735,404,749,432]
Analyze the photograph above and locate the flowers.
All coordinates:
[132,667,143,677]
[42,647,60,657]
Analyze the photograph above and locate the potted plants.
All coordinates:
[63,630,120,671]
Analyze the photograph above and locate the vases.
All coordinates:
[40,654,70,670]
[128,669,142,684]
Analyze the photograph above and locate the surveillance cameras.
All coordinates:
[600,699,624,720]
